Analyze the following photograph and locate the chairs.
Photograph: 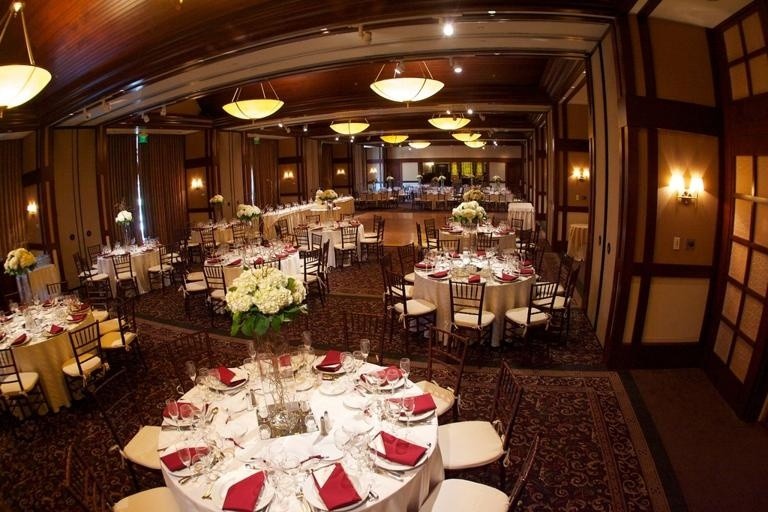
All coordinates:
[384,216,575,359]
[1,282,144,422]
[76,185,409,327]
[409,185,525,213]
[65,310,541,510]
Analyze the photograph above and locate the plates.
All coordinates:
[0,294,91,347]
[418,247,533,284]
[161,331,436,511]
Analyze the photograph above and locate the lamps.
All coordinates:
[328,62,488,150]
[0,1,55,122]
[668,174,705,205]
[220,81,287,124]
